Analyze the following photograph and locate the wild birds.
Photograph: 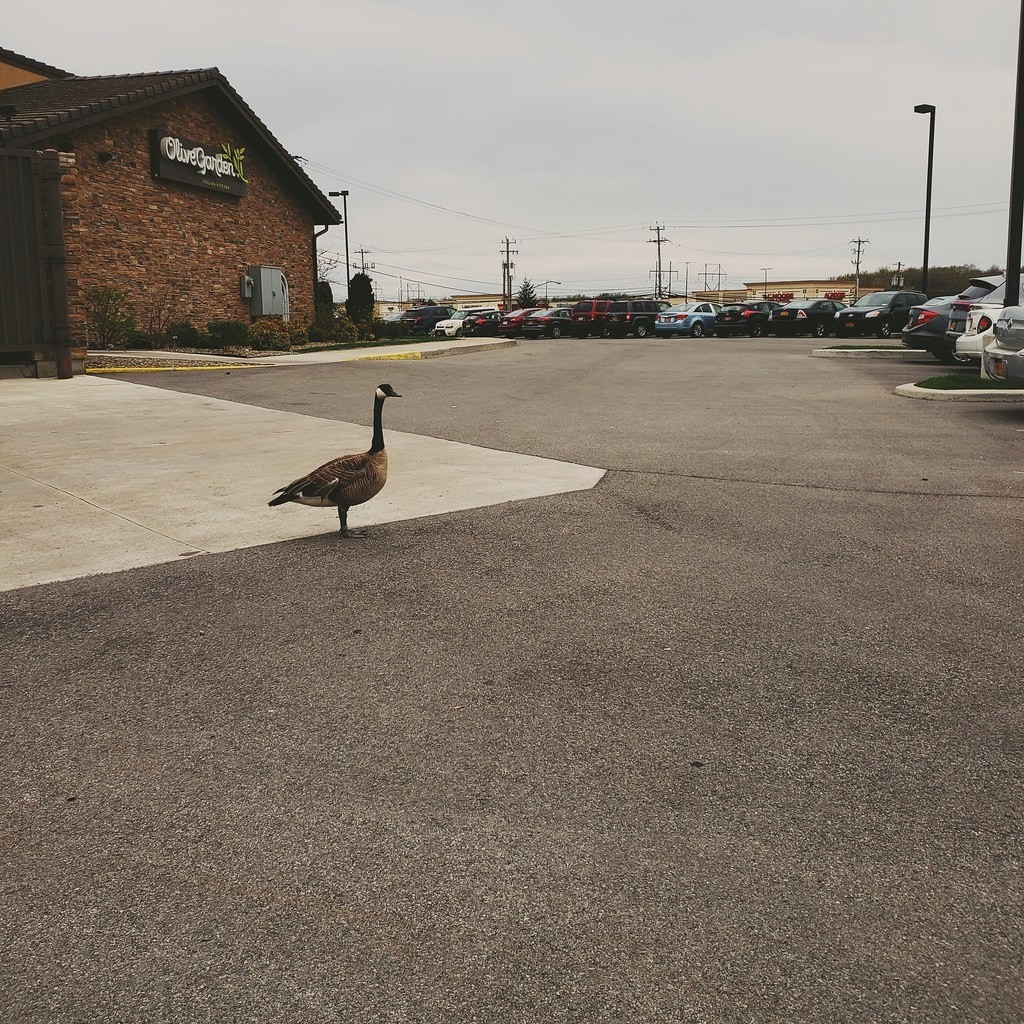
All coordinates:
[267,384,402,538]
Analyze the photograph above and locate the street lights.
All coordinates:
[329,190,350,301]
[914,104,936,297]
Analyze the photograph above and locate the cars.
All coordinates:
[901,274,1024,383]
[655,302,723,338]
[714,298,848,338]
[380,306,574,340]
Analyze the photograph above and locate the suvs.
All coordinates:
[832,289,928,339]
[573,299,672,339]
